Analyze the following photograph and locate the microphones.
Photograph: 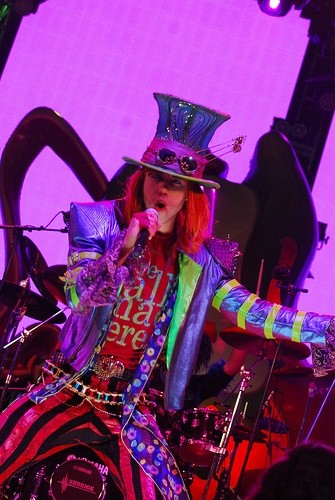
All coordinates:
[262,388,276,409]
[273,263,290,279]
[133,208,158,258]
[61,210,71,223]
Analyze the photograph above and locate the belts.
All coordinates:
[89,355,136,382]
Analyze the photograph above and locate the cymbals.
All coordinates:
[0,280,66,325]
[229,423,267,444]
[21,234,59,305]
[42,264,69,309]
[219,326,311,361]
[271,367,313,378]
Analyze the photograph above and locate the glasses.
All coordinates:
[146,147,198,173]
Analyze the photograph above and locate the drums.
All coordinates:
[176,408,226,466]
[149,387,165,414]
[48,457,105,500]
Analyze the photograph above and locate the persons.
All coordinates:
[0,140,334,500]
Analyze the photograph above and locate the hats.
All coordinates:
[122,91,233,189]
